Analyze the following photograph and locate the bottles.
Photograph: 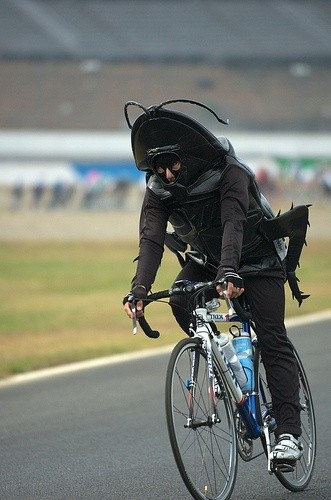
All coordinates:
[230,325,253,393]
[214,333,247,388]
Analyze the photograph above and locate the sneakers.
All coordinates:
[273,433,304,459]
[208,375,223,397]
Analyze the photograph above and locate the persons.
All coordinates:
[122,145,302,463]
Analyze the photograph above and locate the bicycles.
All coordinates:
[129,278,317,500]
